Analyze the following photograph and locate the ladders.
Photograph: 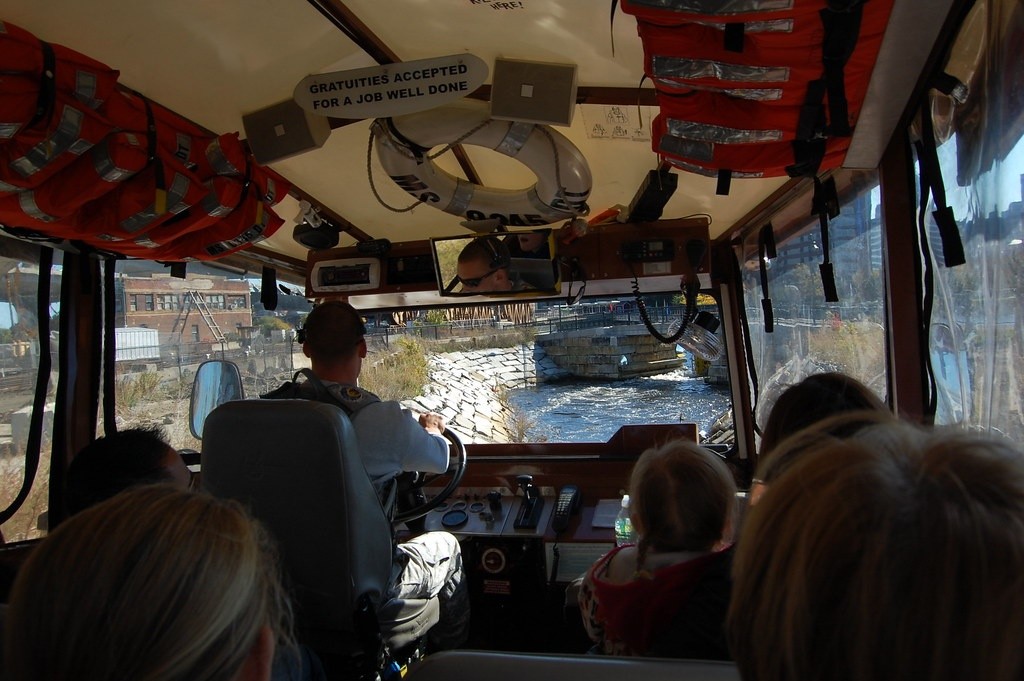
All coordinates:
[189,291,227,343]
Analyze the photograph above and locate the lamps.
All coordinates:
[669,310,723,362]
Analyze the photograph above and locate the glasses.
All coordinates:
[457,267,507,287]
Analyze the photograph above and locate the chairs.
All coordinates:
[200,400,442,681]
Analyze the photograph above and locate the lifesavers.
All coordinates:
[371,97,594,229]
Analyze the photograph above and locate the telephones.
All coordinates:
[552,483,583,534]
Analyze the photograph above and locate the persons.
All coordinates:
[517,233,550,258]
[576,438,744,663]
[758,370,888,471]
[456,235,538,293]
[284,297,470,638]
[6,484,273,680]
[727,408,1024,680]
[59,427,199,511]
